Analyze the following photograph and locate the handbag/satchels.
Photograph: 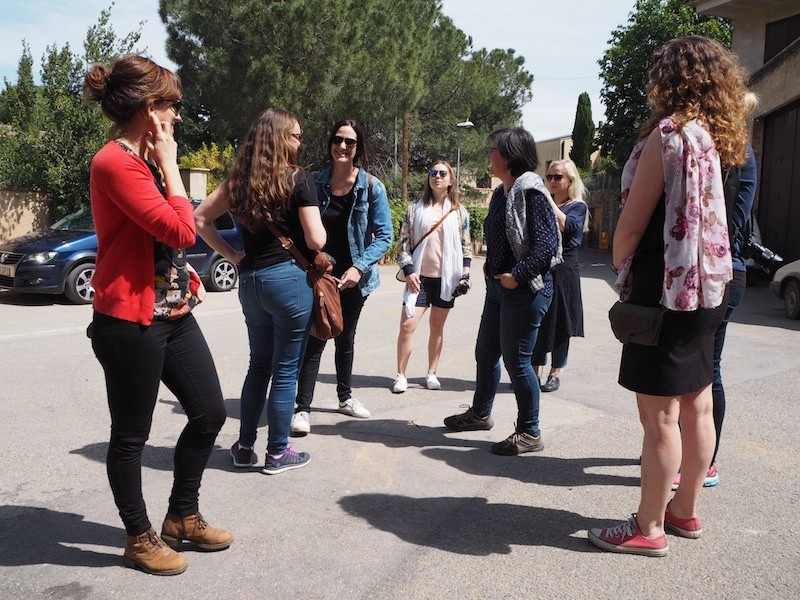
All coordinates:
[395,269,406,282]
[608,300,669,348]
[308,254,344,339]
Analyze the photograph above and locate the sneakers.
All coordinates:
[425,372,441,390]
[672,461,720,490]
[392,373,408,392]
[444,404,494,431]
[160,511,235,550]
[588,514,669,556]
[123,528,189,576]
[664,500,703,539]
[290,411,310,433]
[230,441,254,467]
[262,441,312,474]
[491,421,544,456]
[338,399,370,418]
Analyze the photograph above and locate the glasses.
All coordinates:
[331,136,358,147]
[158,97,182,116]
[489,148,498,153]
[429,169,447,178]
[545,174,563,182]
[290,130,304,144]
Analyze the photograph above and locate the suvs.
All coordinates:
[0,199,243,305]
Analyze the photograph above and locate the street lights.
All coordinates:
[456,121,474,201]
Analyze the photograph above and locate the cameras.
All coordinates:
[451,284,467,297]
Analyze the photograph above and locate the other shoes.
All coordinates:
[541,373,560,392]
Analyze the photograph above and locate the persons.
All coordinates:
[392,160,472,393]
[289,120,394,434]
[442,127,564,455]
[83,55,233,577]
[193,106,326,475]
[508,159,593,392]
[587,35,760,557]
